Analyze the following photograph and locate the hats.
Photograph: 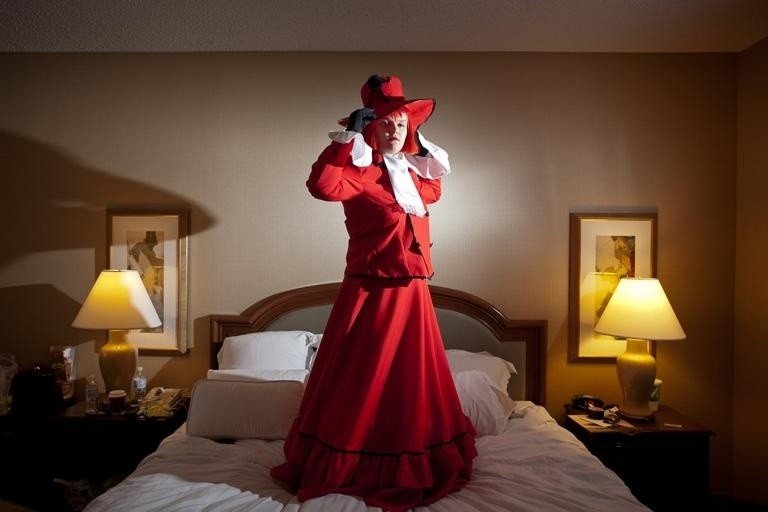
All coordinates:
[337,75,436,156]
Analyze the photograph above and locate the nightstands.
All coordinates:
[50,389,191,484]
[564,405,718,512]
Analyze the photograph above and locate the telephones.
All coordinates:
[143,386,185,408]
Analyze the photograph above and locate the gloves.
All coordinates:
[346,106,377,134]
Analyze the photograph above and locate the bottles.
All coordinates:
[84,374,98,415]
[133,365,147,405]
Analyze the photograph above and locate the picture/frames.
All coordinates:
[568,212,658,362]
[106,208,188,356]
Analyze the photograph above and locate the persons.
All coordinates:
[270,74,477,512]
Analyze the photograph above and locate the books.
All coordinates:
[567,414,639,436]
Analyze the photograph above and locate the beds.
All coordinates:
[84,283,654,512]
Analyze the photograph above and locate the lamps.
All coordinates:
[71,268,163,404]
[593,277,687,421]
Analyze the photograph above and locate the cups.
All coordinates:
[109,390,127,417]
[649,377,662,413]
